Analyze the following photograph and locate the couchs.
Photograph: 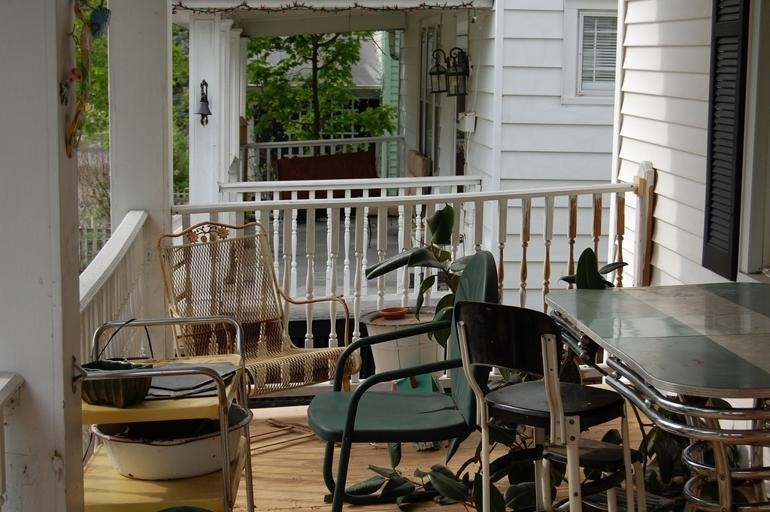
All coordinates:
[277,150,389,223]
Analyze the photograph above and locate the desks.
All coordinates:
[544,282,769,512]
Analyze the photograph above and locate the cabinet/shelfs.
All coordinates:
[78,316,254,512]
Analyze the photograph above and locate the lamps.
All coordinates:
[193,79,212,126]
[429,47,474,97]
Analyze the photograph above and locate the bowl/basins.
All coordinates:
[89,404,253,482]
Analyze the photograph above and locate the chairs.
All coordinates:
[369,151,432,248]
[156,223,357,512]
[305,251,498,510]
[453,299,647,511]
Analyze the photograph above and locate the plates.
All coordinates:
[379,306,408,320]
